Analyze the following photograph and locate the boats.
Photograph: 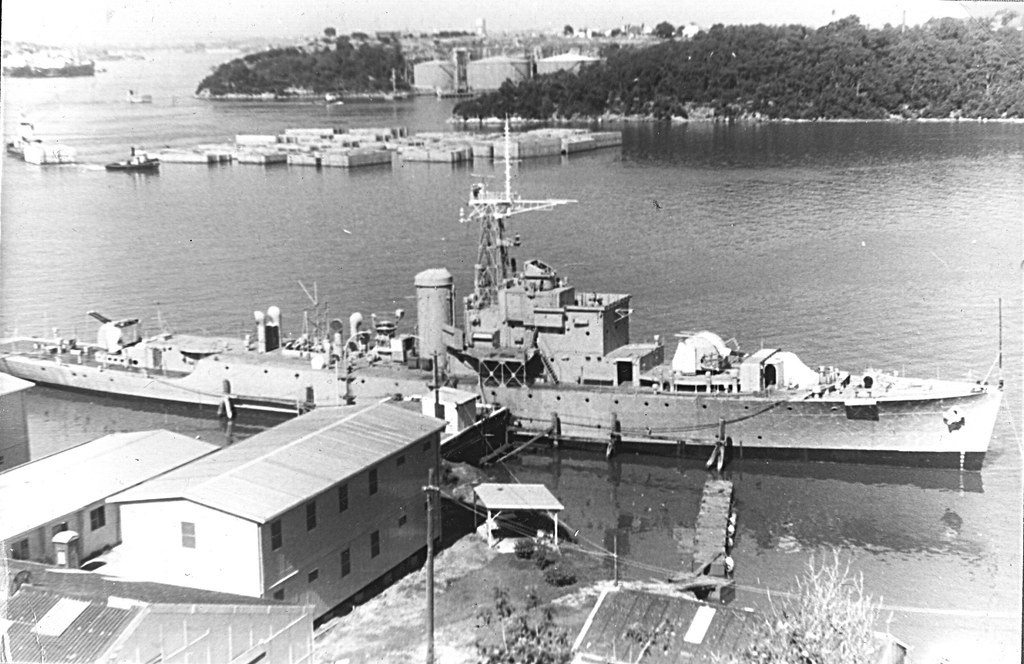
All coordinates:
[131,93,152,104]
[472,482,565,540]
[105,153,160,171]
[1,111,1005,472]
[324,92,344,102]
